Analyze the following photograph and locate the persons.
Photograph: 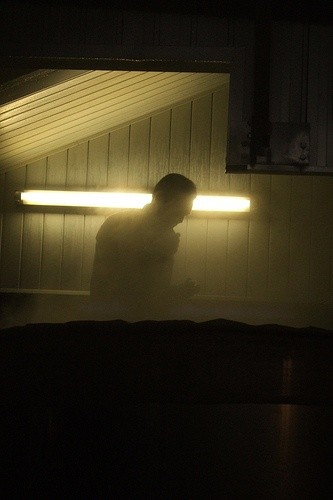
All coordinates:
[84,174,201,322]
